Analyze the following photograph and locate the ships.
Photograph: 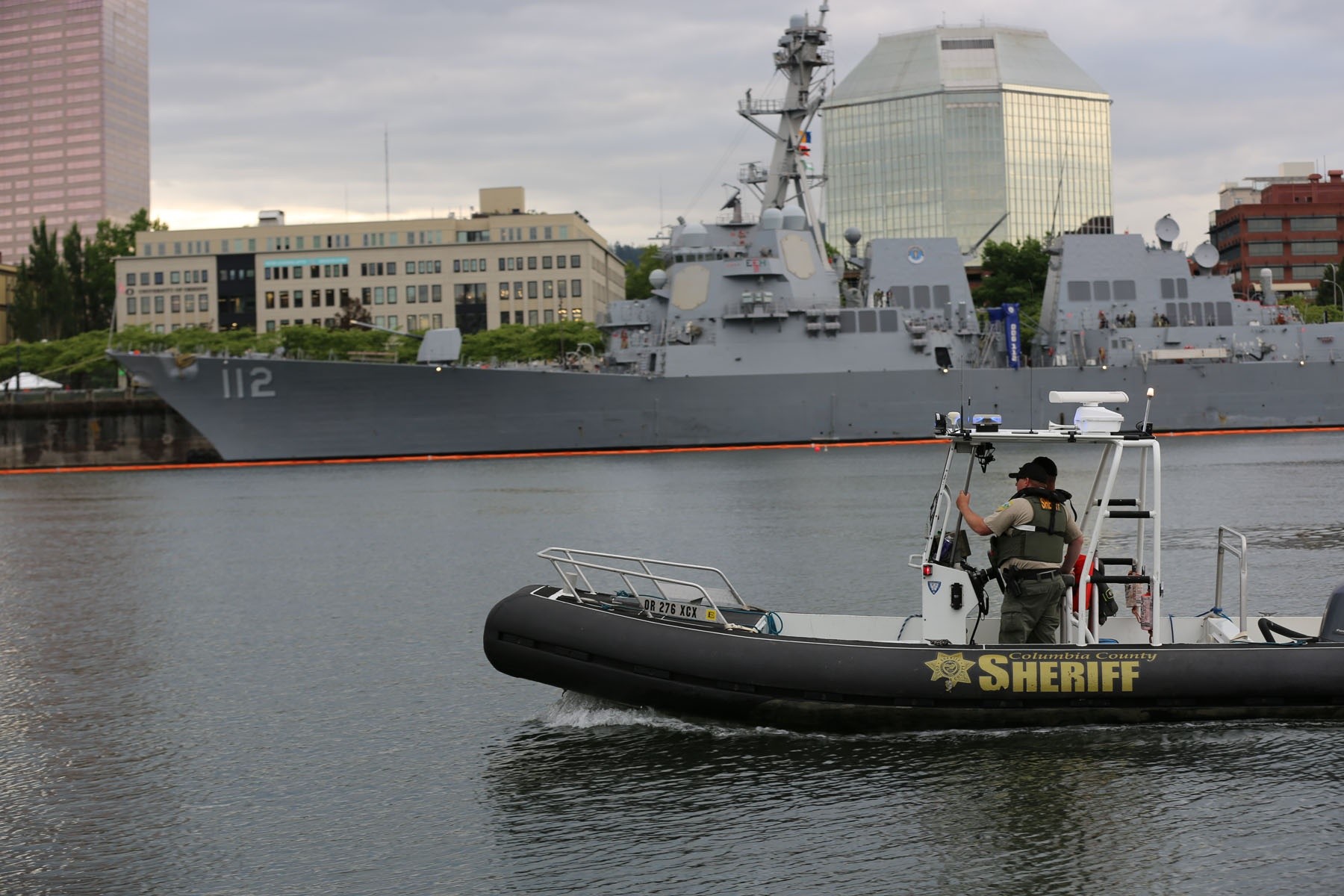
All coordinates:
[108,0,1343,464]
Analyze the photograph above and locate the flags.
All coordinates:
[801,160,814,170]
[799,131,811,143]
[798,146,810,157]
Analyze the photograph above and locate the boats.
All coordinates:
[479,386,1344,731]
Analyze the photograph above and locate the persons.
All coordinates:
[956,461,1084,645]
[886,289,894,308]
[880,291,887,308]
[1031,456,1078,525]
[521,325,651,377]
[1099,309,1171,329]
[873,288,880,308]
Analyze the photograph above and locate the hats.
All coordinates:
[1019,457,1057,476]
[1009,463,1049,484]
